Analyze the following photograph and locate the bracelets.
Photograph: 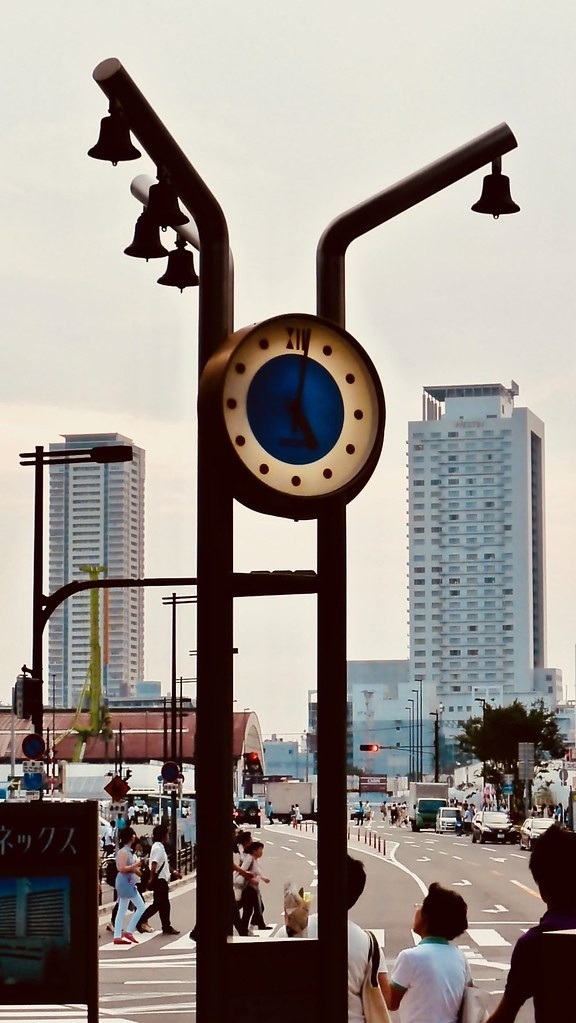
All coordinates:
[174,870,176,873]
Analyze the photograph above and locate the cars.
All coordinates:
[520,818,558,851]
[471,810,517,844]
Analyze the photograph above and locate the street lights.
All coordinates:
[473,697,487,788]
[413,678,423,783]
[405,707,411,781]
[428,712,439,783]
[412,689,419,782]
[408,699,415,781]
[18,444,137,805]
[162,696,191,765]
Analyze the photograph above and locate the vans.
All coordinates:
[435,807,460,835]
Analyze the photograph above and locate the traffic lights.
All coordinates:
[359,744,380,752]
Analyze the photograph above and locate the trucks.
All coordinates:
[406,780,449,833]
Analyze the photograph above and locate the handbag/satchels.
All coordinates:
[234,859,254,889]
[461,951,490,1023]
[141,867,158,891]
[362,930,392,1023]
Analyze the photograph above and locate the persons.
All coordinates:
[355,801,363,826]
[118,814,137,848]
[274,856,391,1023]
[105,821,116,856]
[482,804,508,812]
[364,801,371,821]
[391,801,416,828]
[487,826,576,1023]
[266,802,274,824]
[533,803,569,826]
[128,803,153,825]
[106,825,182,945]
[391,883,474,1023]
[382,801,387,821]
[233,823,270,937]
[290,804,302,824]
[450,797,475,836]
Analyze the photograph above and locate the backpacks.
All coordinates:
[105,859,118,887]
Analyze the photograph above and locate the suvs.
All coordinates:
[236,799,262,828]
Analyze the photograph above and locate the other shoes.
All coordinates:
[162,926,180,934]
[251,920,259,926]
[141,923,155,933]
[258,926,273,930]
[124,932,139,943]
[137,925,144,933]
[106,922,115,933]
[114,938,132,944]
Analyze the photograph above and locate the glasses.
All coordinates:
[413,904,424,909]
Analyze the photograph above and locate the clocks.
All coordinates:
[198,313,388,522]
